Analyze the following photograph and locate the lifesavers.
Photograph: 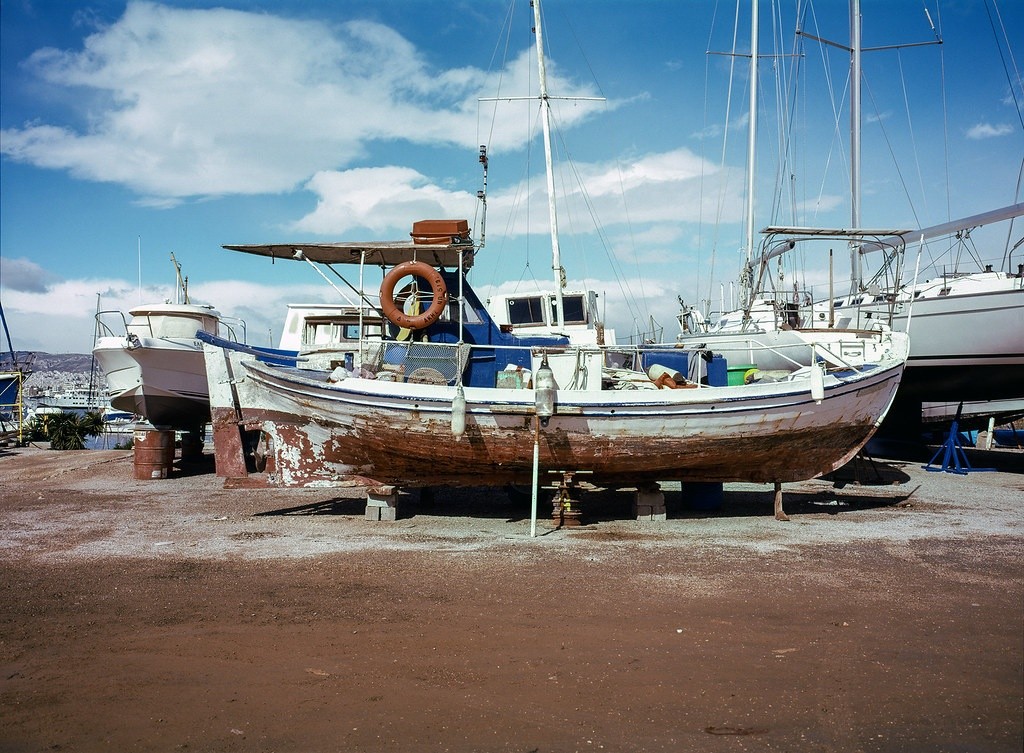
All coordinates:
[379,259,448,330]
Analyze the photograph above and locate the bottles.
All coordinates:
[451,396,466,442]
[811,367,825,405]
[535,360,554,416]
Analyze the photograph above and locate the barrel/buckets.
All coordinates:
[134,429,175,479]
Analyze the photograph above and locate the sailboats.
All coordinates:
[0,0,1024,500]
